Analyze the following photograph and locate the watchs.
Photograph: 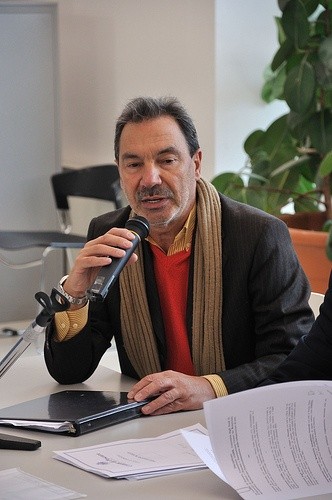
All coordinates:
[58,274,88,304]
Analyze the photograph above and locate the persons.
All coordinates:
[257,269,332,387]
[46,97,314,416]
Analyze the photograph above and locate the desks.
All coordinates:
[0,354,332,500]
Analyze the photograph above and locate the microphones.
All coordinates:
[87,216,150,303]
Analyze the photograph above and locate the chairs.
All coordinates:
[0,163,121,315]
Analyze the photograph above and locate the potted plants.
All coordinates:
[209,0,332,296]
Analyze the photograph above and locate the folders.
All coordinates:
[0,389,163,437]
